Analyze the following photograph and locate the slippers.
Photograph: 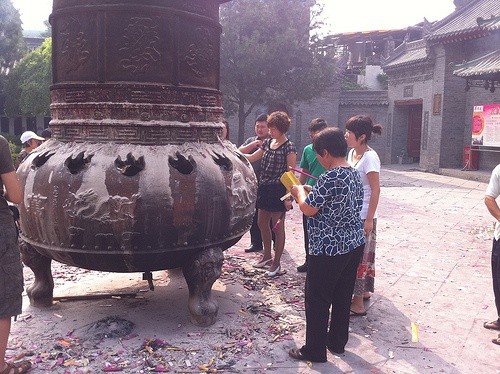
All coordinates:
[483,320,500,331]
[350,310,367,316]
[492,338,500,345]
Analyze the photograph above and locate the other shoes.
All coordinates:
[289,348,306,361]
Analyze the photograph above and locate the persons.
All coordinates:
[238,114,278,252]
[343,115,382,316]
[17,132,46,169]
[290,127,366,362]
[244,111,297,278]
[484,163,500,347]
[0,134,32,374]
[293,118,328,273]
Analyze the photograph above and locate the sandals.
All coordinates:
[1,360,31,374]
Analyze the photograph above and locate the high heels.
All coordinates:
[264,264,281,277]
[252,258,273,268]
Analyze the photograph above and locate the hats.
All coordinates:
[20,131,45,144]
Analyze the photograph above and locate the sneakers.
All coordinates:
[297,264,307,272]
[244,245,263,252]
